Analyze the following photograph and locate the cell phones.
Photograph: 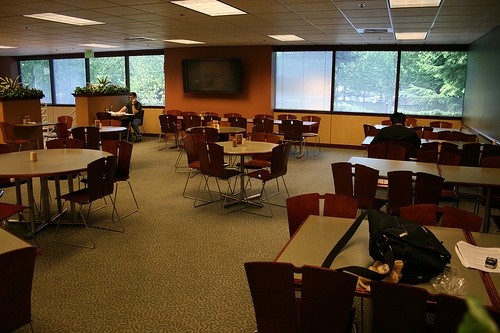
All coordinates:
[485,257,498,269]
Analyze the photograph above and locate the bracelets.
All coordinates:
[132,104,135,105]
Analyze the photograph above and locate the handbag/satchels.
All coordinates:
[364,209,452,285]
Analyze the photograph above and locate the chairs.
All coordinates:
[368,142,407,160]
[285,193,357,240]
[362,118,478,142]
[368,280,499,333]
[0,110,145,249]
[460,142,500,212]
[244,261,357,333]
[331,162,388,212]
[416,141,462,196]
[158,106,321,217]
[400,206,483,234]
[387,171,444,222]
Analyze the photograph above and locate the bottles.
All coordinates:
[233,139,237,147]
[242,138,246,147]
[100,122,102,129]
[391,260,403,282]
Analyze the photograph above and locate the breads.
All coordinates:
[360,259,403,284]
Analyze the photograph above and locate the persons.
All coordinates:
[119,92,144,143]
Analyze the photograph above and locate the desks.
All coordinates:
[67,126,127,134]
[186,126,245,132]
[272,125,500,309]
[176,116,318,158]
[108,111,136,140]
[0,149,114,238]
[13,122,57,150]
[206,140,279,207]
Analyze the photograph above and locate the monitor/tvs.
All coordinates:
[182,58,243,94]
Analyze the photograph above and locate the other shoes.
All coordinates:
[135,138,140,142]
